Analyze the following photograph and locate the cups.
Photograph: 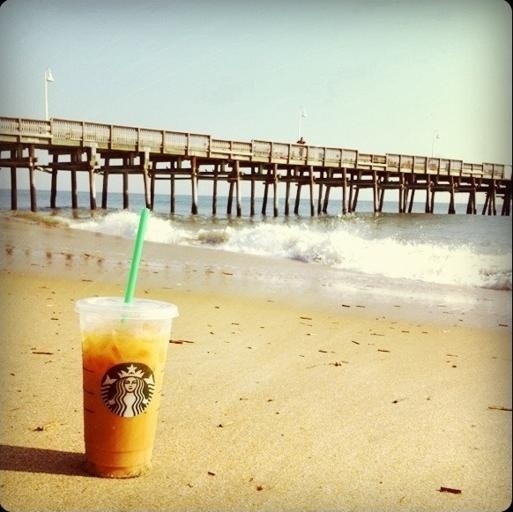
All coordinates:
[76,294,180,480]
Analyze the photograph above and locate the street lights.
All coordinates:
[430,128,441,158]
[296,101,308,145]
[42,66,55,122]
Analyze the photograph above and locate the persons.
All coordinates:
[108,376,148,417]
[297,137,306,160]
